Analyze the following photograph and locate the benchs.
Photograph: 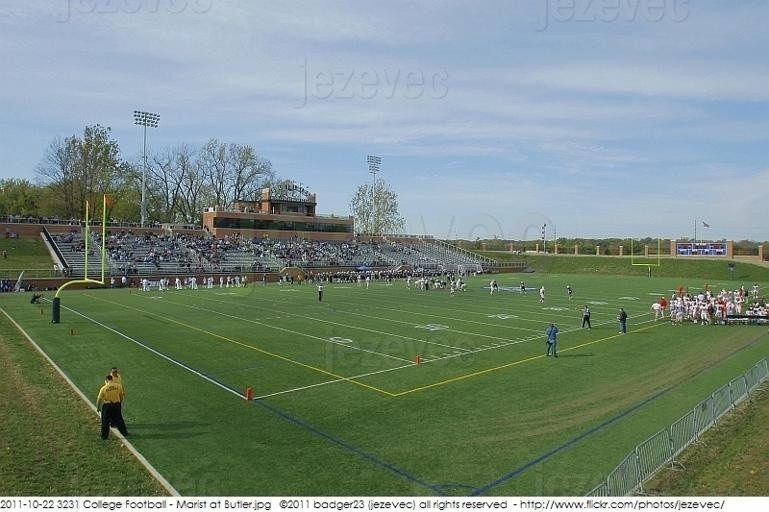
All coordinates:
[50,233,476,277]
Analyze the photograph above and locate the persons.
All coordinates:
[490,279,497,296]
[617,308,627,334]
[649,281,769,326]
[537,286,544,304]
[0,214,479,303]
[544,323,558,358]
[579,305,589,331]
[566,286,573,300]
[96,375,129,440]
[520,281,527,295]
[103,367,126,428]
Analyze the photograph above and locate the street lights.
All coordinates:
[131,110,162,228]
[541,223,548,253]
[367,154,381,234]
